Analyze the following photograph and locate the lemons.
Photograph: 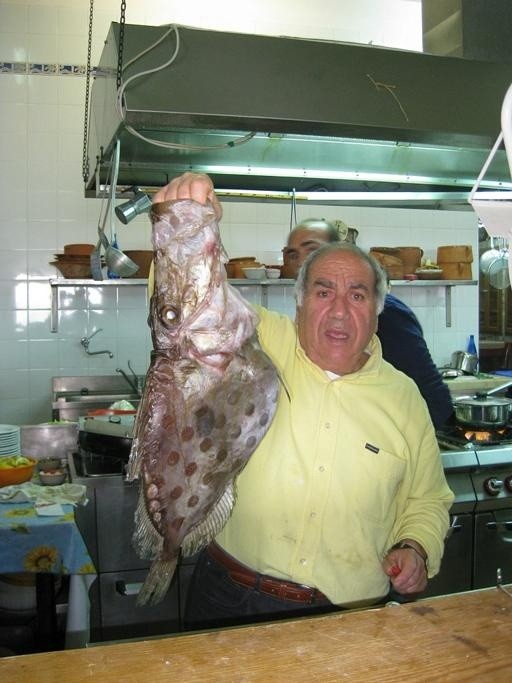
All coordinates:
[114,184,157,223]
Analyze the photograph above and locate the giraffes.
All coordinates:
[432,423,512,452]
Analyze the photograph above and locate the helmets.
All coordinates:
[0,424,22,461]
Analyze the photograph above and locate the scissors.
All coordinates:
[97,153,113,250]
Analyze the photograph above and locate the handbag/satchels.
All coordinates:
[392,542,414,550]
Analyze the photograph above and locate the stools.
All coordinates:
[206,541,327,606]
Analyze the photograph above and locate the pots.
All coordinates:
[449,391,512,428]
[437,350,477,377]
[21,421,80,461]
[78,429,135,458]
[369,245,422,279]
[80,407,139,439]
[223,254,262,278]
[479,235,503,276]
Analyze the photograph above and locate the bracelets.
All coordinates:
[1,457,37,486]
[436,242,474,281]
[415,269,443,279]
[40,469,67,485]
[266,269,281,278]
[39,457,61,470]
[49,242,139,278]
[242,267,266,278]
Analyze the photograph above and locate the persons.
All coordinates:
[284,217,457,431]
[150,171,457,632]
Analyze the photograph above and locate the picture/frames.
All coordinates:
[0,482,92,652]
[0,579,512,681]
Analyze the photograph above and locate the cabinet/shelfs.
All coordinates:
[467,334,478,371]
